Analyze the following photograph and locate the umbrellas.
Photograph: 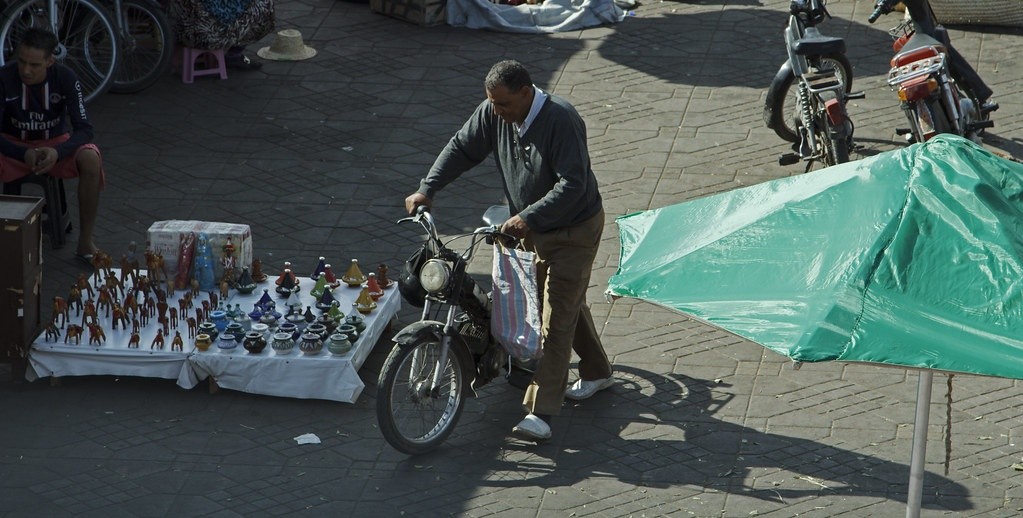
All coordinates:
[603,134,1023,518]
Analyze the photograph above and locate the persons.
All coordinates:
[0,30,105,265]
[403,60,614,439]
[166,0,275,71]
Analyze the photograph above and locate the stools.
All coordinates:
[2,175,73,249]
[179,47,228,83]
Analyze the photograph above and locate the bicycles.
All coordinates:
[0,0,175,113]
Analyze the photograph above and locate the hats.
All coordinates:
[256,30,318,61]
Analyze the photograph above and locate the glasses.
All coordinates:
[511,130,520,160]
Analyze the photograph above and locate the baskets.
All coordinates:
[889,19,915,54]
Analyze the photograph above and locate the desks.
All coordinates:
[0,194,46,349]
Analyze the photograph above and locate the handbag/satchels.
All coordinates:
[491,224,544,360]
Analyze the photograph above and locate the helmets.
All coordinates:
[397,248,437,307]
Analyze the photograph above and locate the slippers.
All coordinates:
[74,244,110,269]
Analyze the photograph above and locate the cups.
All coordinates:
[33,138,48,174]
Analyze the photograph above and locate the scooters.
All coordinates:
[868,0,1000,157]
[373,204,534,457]
[763,0,866,179]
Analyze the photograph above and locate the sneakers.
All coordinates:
[512,413,552,440]
[564,374,615,400]
